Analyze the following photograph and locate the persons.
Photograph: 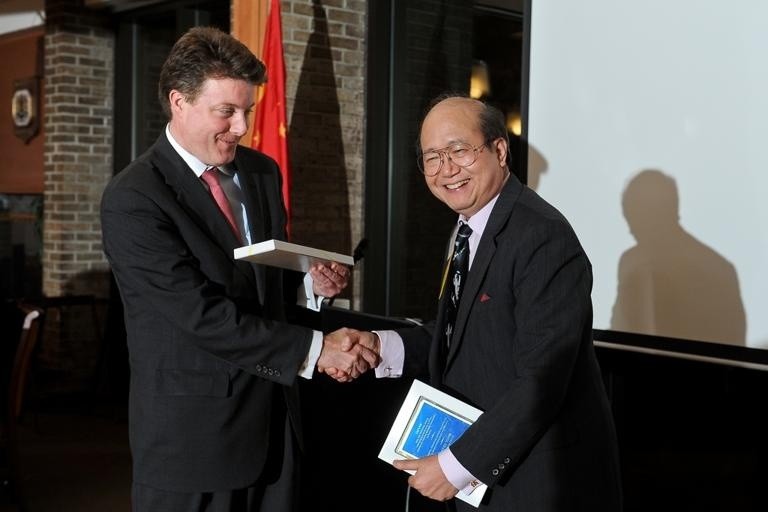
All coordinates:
[321,89,625,511]
[100,25,382,512]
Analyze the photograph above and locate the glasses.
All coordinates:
[416,139,494,177]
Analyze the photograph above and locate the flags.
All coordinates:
[247,1,292,240]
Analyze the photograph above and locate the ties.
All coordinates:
[442,223,473,352]
[201,166,244,247]
